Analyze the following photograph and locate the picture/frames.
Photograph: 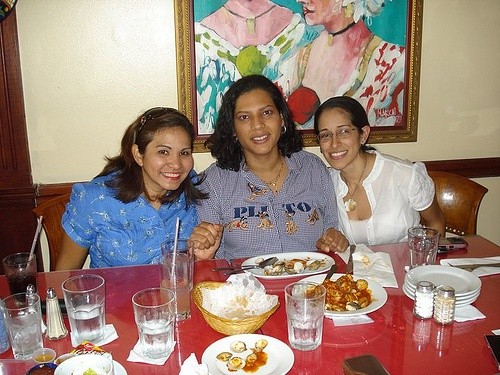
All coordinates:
[173,0,424,153]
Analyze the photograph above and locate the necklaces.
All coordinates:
[242,157,283,193]
[345,158,368,212]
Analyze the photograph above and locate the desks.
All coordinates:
[0,233,500,375]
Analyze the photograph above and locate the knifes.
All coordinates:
[344,245,356,274]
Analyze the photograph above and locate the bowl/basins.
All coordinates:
[32,347,56,365]
[54,354,115,375]
[24,362,58,375]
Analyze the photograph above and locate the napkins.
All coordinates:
[351,243,399,289]
[178,352,208,375]
[14,306,48,341]
[325,314,376,327]
[126,336,177,366]
[70,323,120,349]
[454,304,487,323]
[440,255,500,277]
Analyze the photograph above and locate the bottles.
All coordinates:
[44,287,69,340]
[413,281,435,320]
[25,285,47,334]
[434,285,456,325]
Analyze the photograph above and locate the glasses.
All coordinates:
[133,107,180,143]
[317,127,356,143]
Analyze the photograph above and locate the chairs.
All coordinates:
[32,191,73,272]
[417,171,490,237]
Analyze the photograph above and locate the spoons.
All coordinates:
[212,257,278,276]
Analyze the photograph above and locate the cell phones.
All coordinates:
[437,237,468,249]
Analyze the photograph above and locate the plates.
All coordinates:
[291,274,388,317]
[241,252,335,281]
[113,360,128,375]
[201,333,294,375]
[402,264,482,306]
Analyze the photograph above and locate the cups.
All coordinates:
[3,252,38,308]
[0,293,44,360]
[131,287,177,359]
[284,281,326,351]
[62,274,106,345]
[407,226,439,269]
[157,253,190,322]
[162,237,195,292]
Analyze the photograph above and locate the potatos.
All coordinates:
[307,274,371,311]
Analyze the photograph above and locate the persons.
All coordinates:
[55,107,210,271]
[315,96,446,246]
[186,75,349,260]
